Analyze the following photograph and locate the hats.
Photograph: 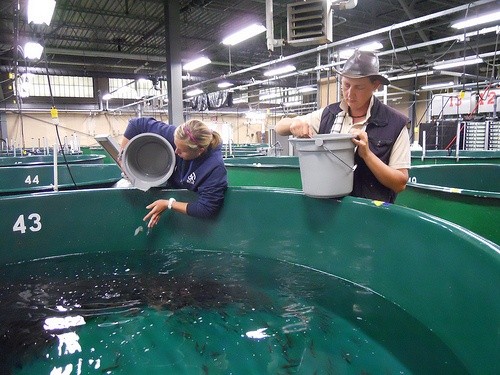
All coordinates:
[332,50,391,85]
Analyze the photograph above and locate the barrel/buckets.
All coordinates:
[288,134,357,198]
[122,133,176,192]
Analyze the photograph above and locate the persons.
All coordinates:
[274,51,411,204]
[117,117,228,228]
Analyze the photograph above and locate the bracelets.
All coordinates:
[168,198,176,209]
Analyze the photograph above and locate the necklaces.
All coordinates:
[349,113,367,118]
